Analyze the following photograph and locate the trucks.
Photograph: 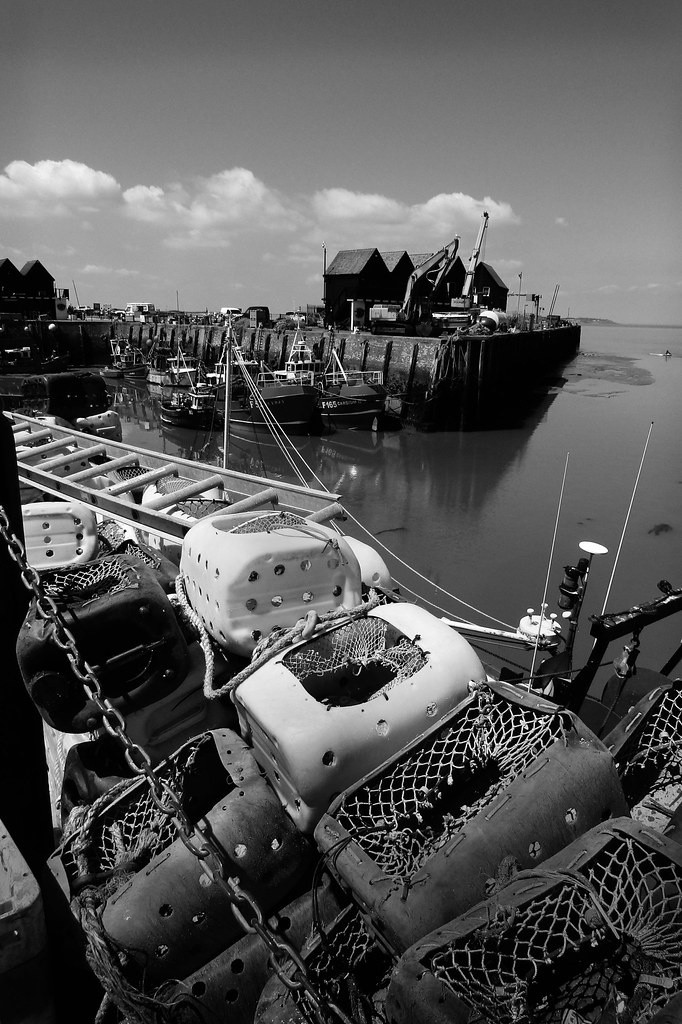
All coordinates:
[124,303,155,314]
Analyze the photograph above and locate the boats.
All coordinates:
[97,308,390,433]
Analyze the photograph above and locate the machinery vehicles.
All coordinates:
[369,211,497,338]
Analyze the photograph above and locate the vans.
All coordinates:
[220,306,245,320]
